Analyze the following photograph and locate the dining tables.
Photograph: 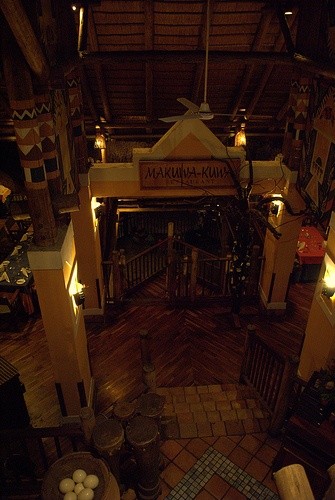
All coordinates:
[0,224,39,316]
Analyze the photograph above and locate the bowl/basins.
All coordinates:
[42,452,111,500]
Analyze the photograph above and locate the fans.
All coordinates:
[158,0,276,123]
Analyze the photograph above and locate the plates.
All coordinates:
[17,279,26,285]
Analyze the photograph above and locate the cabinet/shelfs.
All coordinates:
[271,409,334,497]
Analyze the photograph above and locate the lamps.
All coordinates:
[84,116,109,150]
[234,116,248,146]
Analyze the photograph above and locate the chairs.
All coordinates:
[4,216,27,239]
[0,227,16,261]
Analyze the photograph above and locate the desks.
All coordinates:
[296,224,325,282]
